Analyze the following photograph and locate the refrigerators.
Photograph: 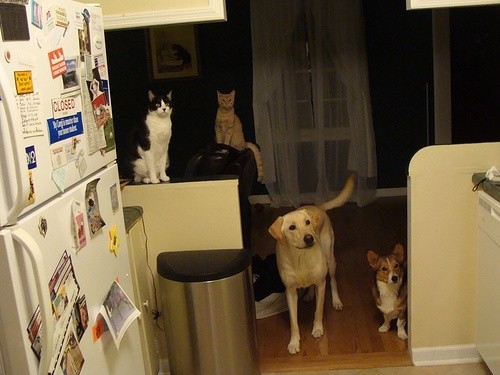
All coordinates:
[0,0,147,375]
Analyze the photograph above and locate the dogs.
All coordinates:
[267,173,356,356]
[365,243,408,341]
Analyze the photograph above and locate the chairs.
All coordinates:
[184,143,258,249]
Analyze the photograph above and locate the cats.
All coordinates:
[126,88,174,184]
[214,90,264,183]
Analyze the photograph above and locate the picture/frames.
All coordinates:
[144,24,203,83]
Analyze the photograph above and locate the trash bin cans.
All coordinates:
[156,249,258,374]
